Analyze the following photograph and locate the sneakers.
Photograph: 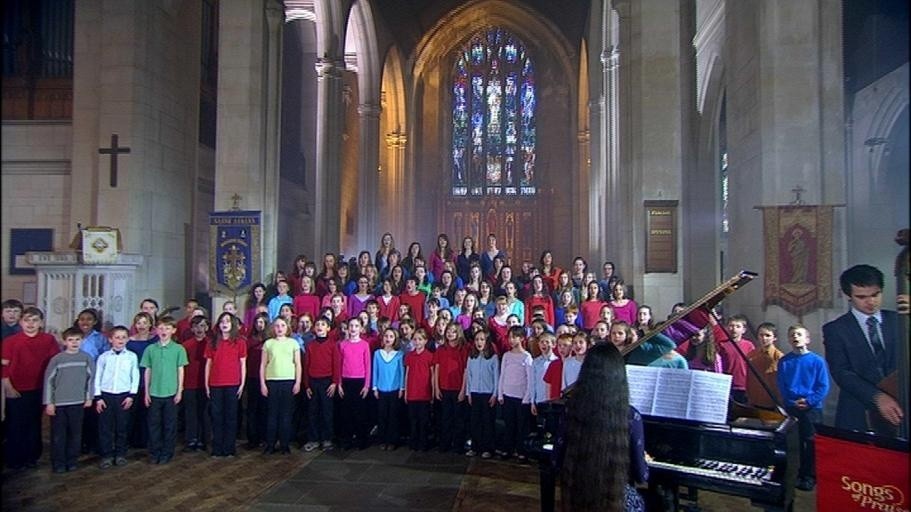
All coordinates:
[796,477,813,491]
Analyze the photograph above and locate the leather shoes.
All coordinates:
[54,456,127,473]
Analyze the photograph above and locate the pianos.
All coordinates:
[532,269,800,511]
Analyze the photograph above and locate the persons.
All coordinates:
[2,299,24,336]
[2,307,61,473]
[139,317,188,466]
[92,326,141,469]
[171,232,785,478]
[777,326,833,491]
[42,326,95,474]
[551,341,650,512]
[73,308,111,360]
[822,265,911,443]
[126,313,159,450]
[788,228,811,291]
[129,298,161,337]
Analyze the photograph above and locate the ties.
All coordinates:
[867,316,887,379]
